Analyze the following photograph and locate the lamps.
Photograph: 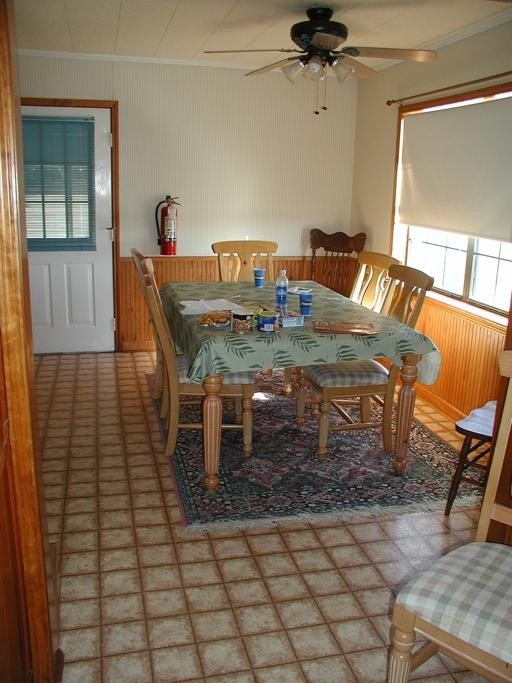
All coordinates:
[282,51,355,113]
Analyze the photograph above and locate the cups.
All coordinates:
[300,289,313,314]
[253,267,267,289]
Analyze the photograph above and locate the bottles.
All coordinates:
[274,267,290,310]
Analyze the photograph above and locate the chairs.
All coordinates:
[445,400,499,516]
[310,228,366,299]
[386,350,512,683]
[145,297,234,418]
[295,264,434,459]
[212,241,278,377]
[283,252,402,419]
[131,248,260,456]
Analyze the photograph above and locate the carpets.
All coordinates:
[147,368,486,536]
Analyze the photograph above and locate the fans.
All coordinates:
[204,8,437,80]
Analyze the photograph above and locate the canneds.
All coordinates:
[232,311,253,335]
[258,311,281,333]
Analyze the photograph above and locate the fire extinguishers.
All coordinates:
[155,195,181,255]
